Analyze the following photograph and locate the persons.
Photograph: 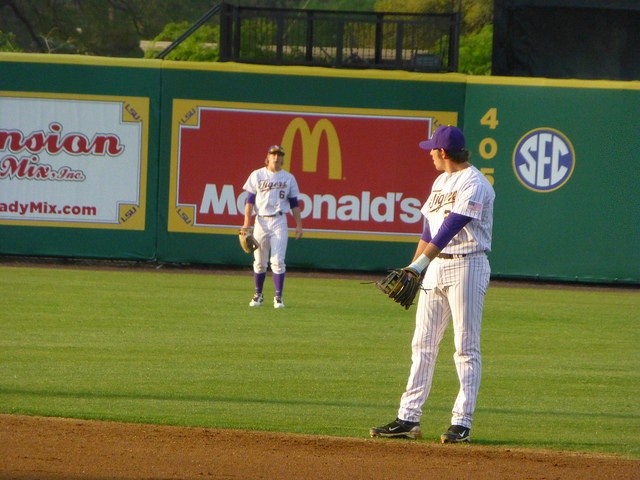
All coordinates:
[239,144,305,310]
[368,123,495,443]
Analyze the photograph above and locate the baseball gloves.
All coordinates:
[239,233,261,254]
[375,268,426,311]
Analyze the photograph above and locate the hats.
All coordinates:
[419,124,465,152]
[268,145,285,155]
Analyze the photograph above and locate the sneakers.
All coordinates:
[273,296,284,308]
[249,293,263,306]
[370,417,421,439]
[441,425,470,443]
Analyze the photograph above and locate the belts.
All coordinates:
[437,253,466,259]
[258,212,282,217]
